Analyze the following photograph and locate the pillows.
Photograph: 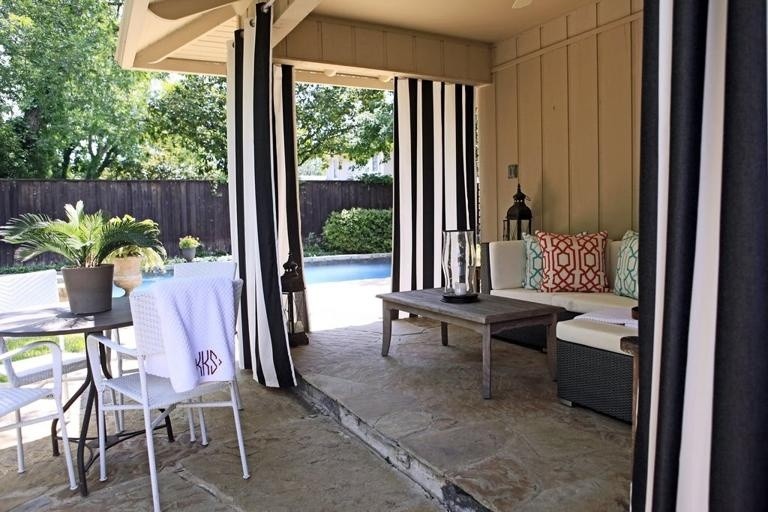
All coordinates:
[488,229,639,301]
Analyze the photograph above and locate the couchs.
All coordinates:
[480,240,640,424]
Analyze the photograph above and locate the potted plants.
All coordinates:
[179,235,200,262]
[0,200,167,316]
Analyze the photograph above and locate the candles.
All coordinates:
[455,282,466,295]
